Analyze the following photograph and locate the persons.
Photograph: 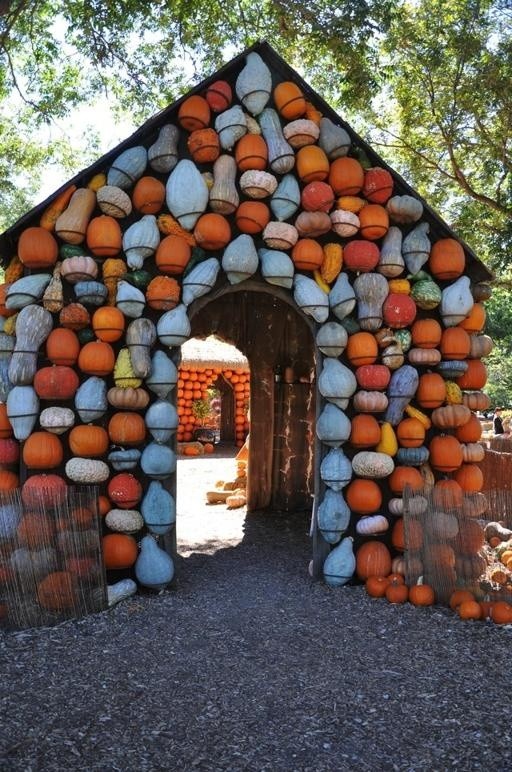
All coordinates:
[492,407,504,434]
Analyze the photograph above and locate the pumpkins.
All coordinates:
[0,49,512,624]
